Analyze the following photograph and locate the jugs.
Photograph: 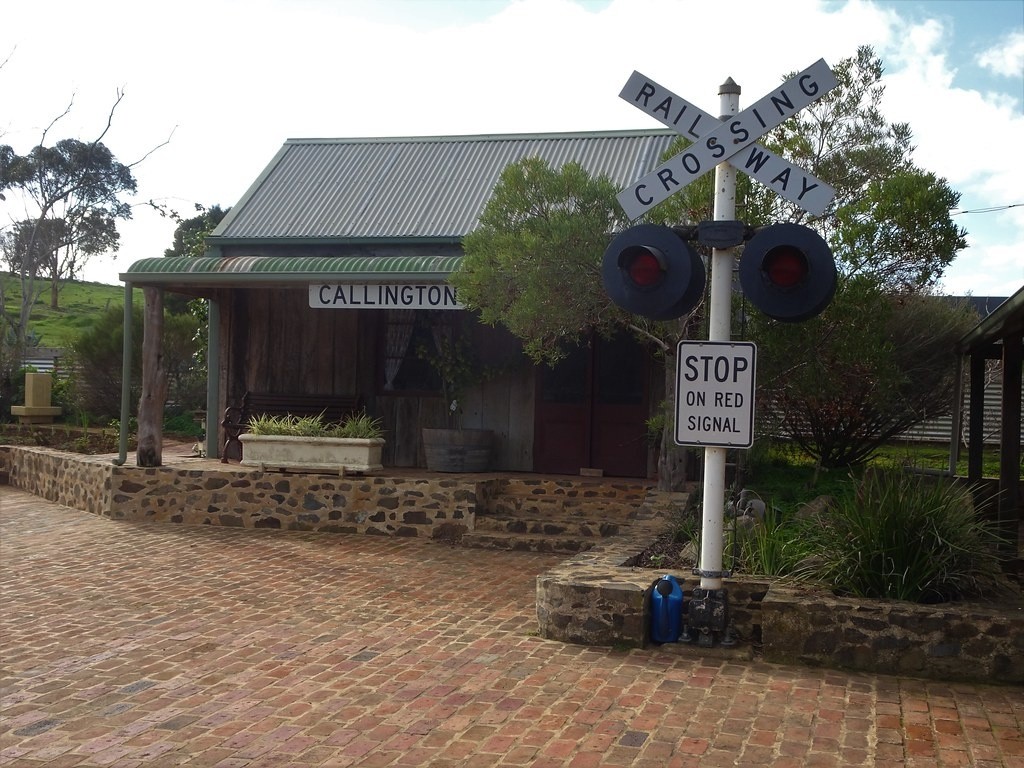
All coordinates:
[649,574,683,643]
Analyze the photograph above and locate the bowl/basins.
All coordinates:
[422,427,494,473]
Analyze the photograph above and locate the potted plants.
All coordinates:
[238,409,388,474]
[412,324,500,472]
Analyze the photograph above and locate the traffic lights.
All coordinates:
[761,244,814,297]
[616,244,669,290]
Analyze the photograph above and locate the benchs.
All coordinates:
[221,393,367,463]
